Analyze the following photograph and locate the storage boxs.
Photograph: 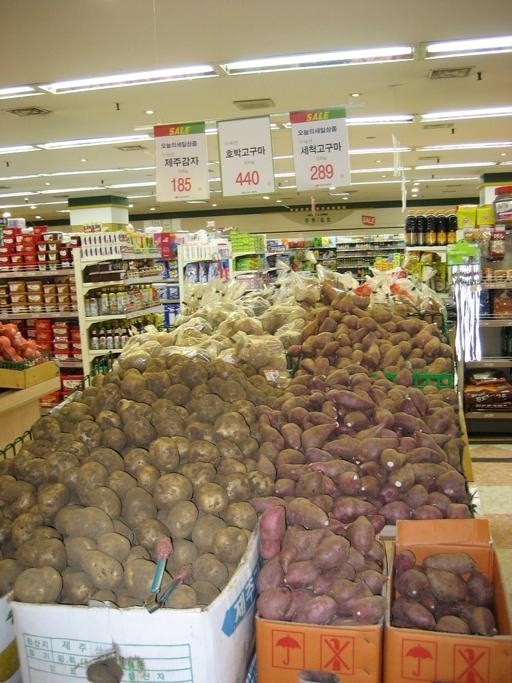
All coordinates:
[0,590,21,682]
[381,519,512,683]
[68,196,129,223]
[255,539,386,683]
[479,180,512,204]
[13,525,260,683]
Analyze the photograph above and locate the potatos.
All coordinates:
[300,671,339,683]
[393,549,499,635]
[89,662,120,682]
[260,368,472,520]
[293,283,454,372]
[0,355,280,607]
[255,497,387,624]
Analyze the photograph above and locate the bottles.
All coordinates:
[416,209,426,245]
[98,329,106,349]
[96,291,101,316]
[437,208,447,245]
[122,286,128,314]
[128,284,136,312]
[427,209,437,245]
[120,328,129,348]
[99,287,108,314]
[89,288,97,315]
[494,184,512,230]
[90,330,98,349]
[84,293,89,316]
[108,287,117,314]
[145,284,152,308]
[106,329,113,348]
[134,284,144,310]
[136,317,144,333]
[447,208,457,243]
[140,284,148,309]
[114,329,120,348]
[117,284,123,314]
[406,209,416,246]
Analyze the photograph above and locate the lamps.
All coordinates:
[222,44,415,75]
[0,82,49,99]
[41,60,218,95]
[38,132,155,149]
[0,143,40,154]
[421,106,512,124]
[284,115,416,128]
[423,33,512,59]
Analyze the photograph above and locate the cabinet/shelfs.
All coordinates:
[72,248,163,388]
[447,228,512,442]
[289,237,337,276]
[338,234,406,280]
[231,235,289,291]
[0,266,81,407]
[147,244,232,333]
